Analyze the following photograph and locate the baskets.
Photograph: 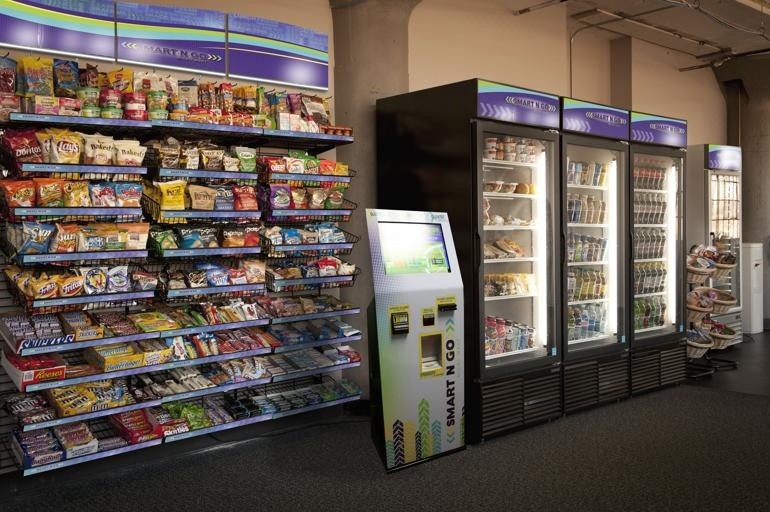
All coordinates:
[686,252,738,359]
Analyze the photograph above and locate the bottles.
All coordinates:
[568,303,604,342]
[633,296,666,331]
[567,232,605,263]
[567,269,606,302]
[634,191,666,224]
[634,227,666,259]
[485,315,534,356]
[633,157,666,190]
[567,193,605,223]
[633,262,667,295]
[567,159,607,188]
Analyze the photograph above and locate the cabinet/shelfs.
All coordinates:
[685,245,738,379]
[0,112,369,478]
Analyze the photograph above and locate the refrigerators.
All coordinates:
[561,95,629,415]
[630,107,686,396]
[376,79,562,445]
[685,143,744,352]
